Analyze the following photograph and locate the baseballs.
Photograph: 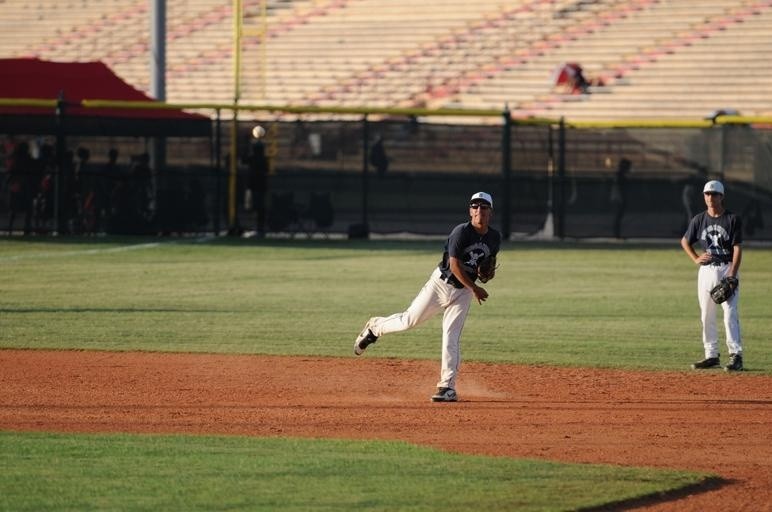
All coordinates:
[253,126,265,138]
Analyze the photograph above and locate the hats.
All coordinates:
[469,190,494,209]
[702,179,726,197]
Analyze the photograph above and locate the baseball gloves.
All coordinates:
[710,277,739,304]
[476,255,496,283]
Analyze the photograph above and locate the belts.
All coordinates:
[701,260,731,268]
[439,270,466,290]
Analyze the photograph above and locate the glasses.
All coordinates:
[469,203,492,211]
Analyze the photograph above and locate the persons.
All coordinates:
[1,136,154,241]
[570,74,593,95]
[242,140,268,237]
[681,180,744,371]
[354,192,501,401]
[610,158,633,240]
[682,165,704,225]
[369,136,390,174]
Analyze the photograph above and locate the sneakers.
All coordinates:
[353,318,378,358]
[725,353,744,373]
[430,386,459,403]
[690,352,723,371]
